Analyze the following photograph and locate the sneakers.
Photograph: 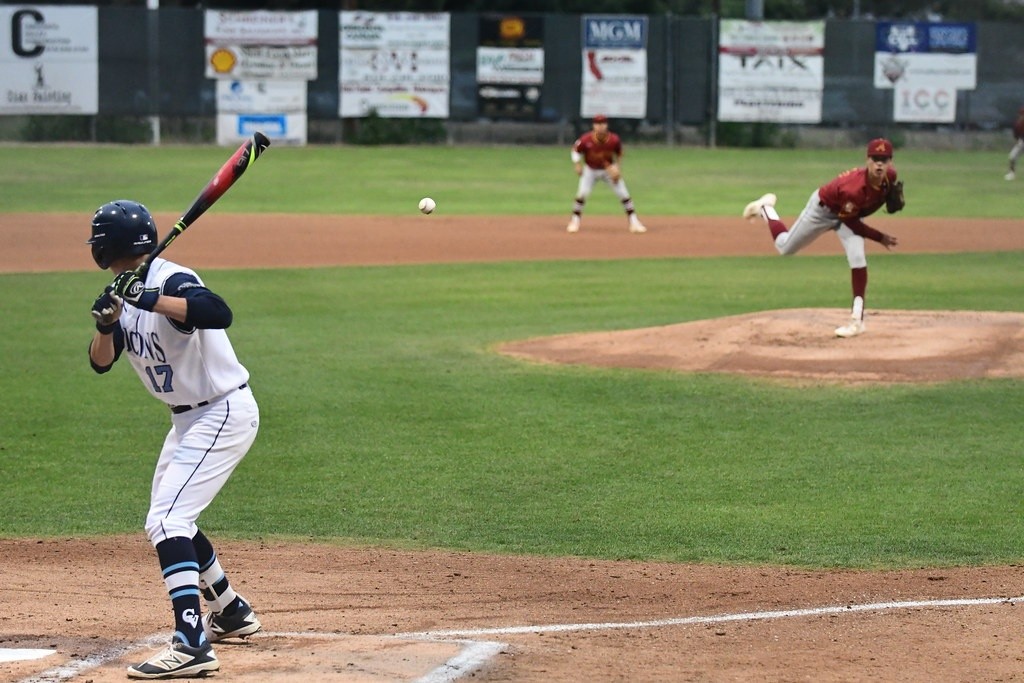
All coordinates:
[743,193,777,218]
[127,637,219,679]
[835,314,865,338]
[201,602,261,643]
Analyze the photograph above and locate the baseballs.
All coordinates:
[418,197,436,215]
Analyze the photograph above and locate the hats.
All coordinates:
[867,138,892,159]
[592,115,607,123]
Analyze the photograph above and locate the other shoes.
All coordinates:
[1004,170,1016,181]
[629,220,646,233]
[566,214,581,232]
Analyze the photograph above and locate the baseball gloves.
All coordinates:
[884,181,905,214]
[606,163,621,184]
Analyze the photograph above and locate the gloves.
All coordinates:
[92,286,121,335]
[114,270,160,312]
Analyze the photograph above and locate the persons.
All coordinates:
[88,200,262,680]
[567,116,647,233]
[1004,107,1024,181]
[742,138,905,338]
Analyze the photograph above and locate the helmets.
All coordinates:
[86,200,158,270]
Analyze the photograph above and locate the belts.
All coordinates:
[168,383,247,414]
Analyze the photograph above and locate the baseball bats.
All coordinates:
[90,130,271,322]
